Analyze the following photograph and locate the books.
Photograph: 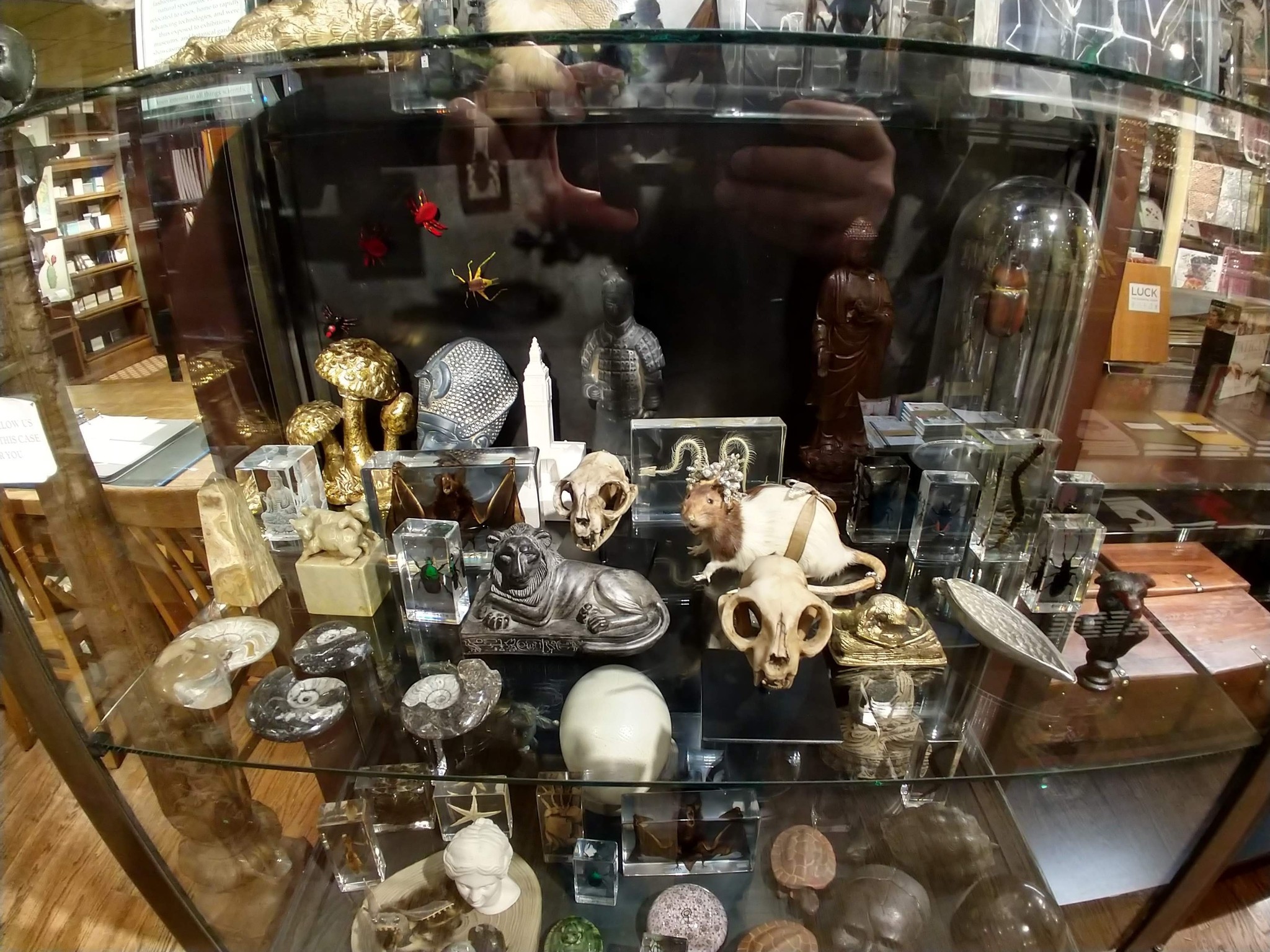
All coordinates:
[172,125,240,200]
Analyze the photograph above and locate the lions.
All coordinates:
[473,522,673,656]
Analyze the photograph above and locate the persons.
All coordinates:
[578,266,665,460]
[168,0,977,486]
[814,212,894,460]
[597,0,672,82]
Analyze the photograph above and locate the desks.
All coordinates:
[0,382,215,581]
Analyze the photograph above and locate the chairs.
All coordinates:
[0,484,273,769]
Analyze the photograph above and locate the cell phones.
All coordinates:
[595,83,853,213]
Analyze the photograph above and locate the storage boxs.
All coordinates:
[1002,540,1270,766]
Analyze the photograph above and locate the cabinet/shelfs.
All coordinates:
[12,87,158,386]
